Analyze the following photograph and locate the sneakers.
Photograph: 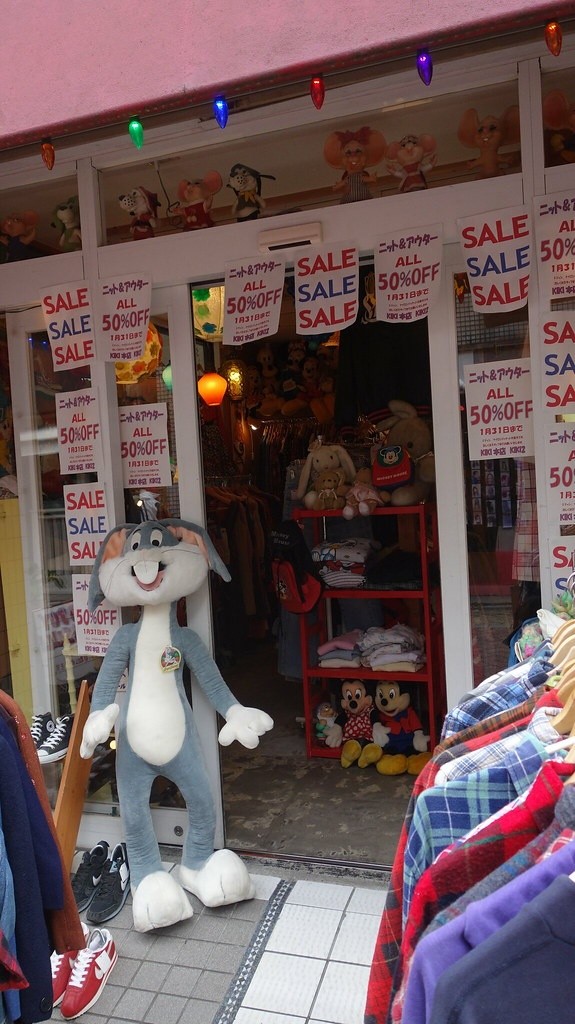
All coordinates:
[29,712,55,749]
[49,922,90,1008]
[86,842,131,924]
[36,712,75,764]
[70,840,111,912]
[60,927,118,1020]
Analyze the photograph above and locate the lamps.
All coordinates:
[191,286,225,344]
[115,321,163,384]
[220,359,248,401]
[197,372,228,422]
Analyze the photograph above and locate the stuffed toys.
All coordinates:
[1,88,575,263]
[80,518,275,934]
[244,341,436,776]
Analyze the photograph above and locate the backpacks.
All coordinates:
[264,519,323,615]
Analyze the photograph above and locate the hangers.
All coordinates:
[260,416,335,444]
[533,571,575,788]
[204,474,280,513]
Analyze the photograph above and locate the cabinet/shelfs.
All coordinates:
[292,499,447,760]
